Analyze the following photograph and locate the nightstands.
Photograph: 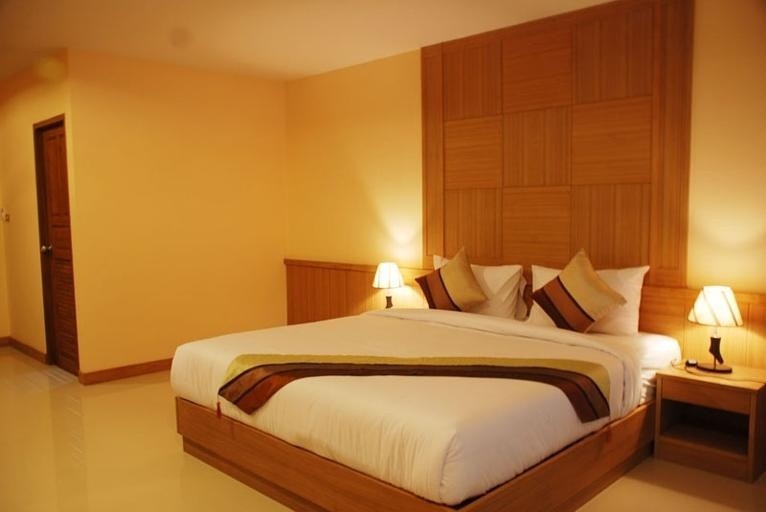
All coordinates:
[654,362,766,484]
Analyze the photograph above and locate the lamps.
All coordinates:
[686,285,743,374]
[373,262,404,309]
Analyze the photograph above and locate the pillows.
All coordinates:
[525,266,651,337]
[414,246,487,311]
[529,249,626,331]
[433,254,523,320]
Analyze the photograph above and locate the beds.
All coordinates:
[170,307,682,512]
